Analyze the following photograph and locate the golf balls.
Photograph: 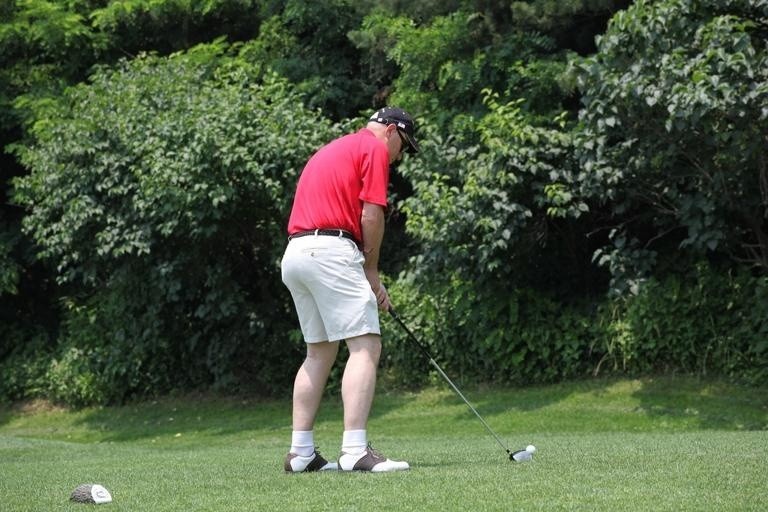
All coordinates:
[525,445,536,454]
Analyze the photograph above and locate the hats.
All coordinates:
[369,105,419,153]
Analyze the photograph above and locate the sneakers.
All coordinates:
[284,447,338,471]
[338,442,409,471]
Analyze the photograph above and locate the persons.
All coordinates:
[279,105,422,473]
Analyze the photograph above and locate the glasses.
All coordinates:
[397,129,410,152]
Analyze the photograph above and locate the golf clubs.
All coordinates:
[388,306,532,462]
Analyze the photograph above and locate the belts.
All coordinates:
[289,228,353,240]
